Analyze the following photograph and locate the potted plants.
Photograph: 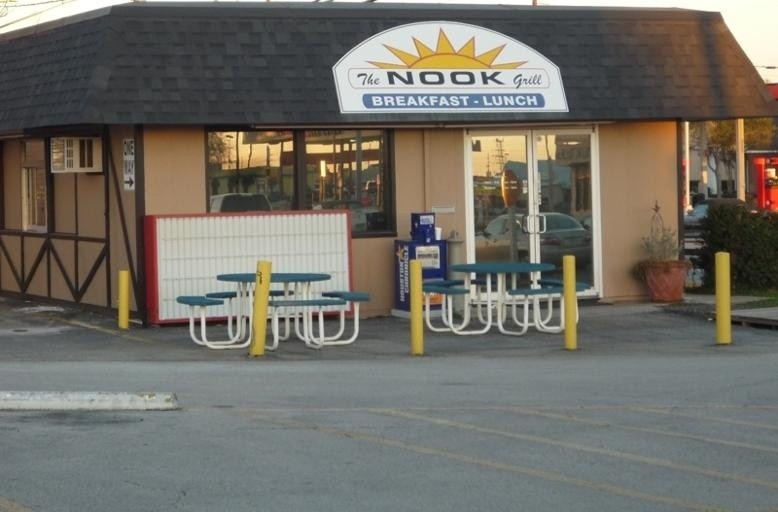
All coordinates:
[634,199,693,305]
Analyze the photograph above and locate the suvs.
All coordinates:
[209,192,272,211]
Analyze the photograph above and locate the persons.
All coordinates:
[692,193,707,213]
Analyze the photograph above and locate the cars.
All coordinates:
[314,200,364,210]
[475,212,591,271]
[265,192,288,209]
[683,196,748,229]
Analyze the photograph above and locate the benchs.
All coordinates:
[170,272,373,353]
[422,260,579,339]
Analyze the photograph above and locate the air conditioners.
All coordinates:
[49,136,104,174]
